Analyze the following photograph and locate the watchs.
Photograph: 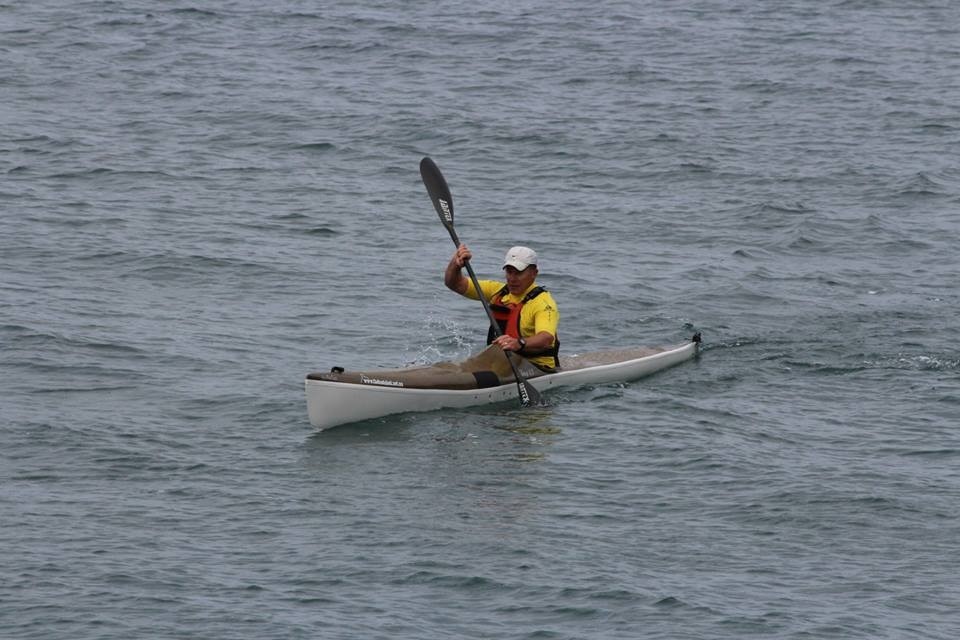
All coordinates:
[518,338,526,350]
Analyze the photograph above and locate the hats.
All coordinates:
[501,245,539,272]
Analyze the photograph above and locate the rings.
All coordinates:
[505,342,508,344]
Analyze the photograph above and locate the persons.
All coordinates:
[444,242,560,382]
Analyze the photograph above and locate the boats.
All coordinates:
[305,320,703,429]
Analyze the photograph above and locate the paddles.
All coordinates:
[417,156,546,405]
